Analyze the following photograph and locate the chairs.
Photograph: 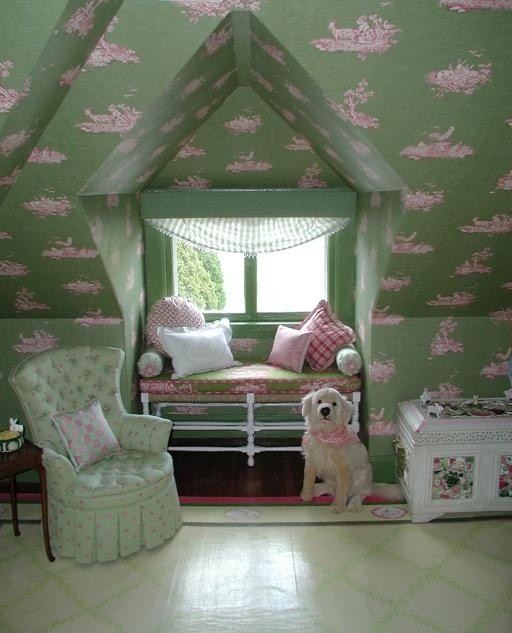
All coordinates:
[9,343,183,563]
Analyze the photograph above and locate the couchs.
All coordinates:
[139,319,363,466]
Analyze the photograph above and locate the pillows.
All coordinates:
[136,348,163,378]
[294,299,353,372]
[267,324,313,374]
[52,401,121,474]
[156,318,243,379]
[335,342,362,375]
[146,295,205,354]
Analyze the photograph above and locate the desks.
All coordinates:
[0,431,55,564]
[395,397,512,524]
